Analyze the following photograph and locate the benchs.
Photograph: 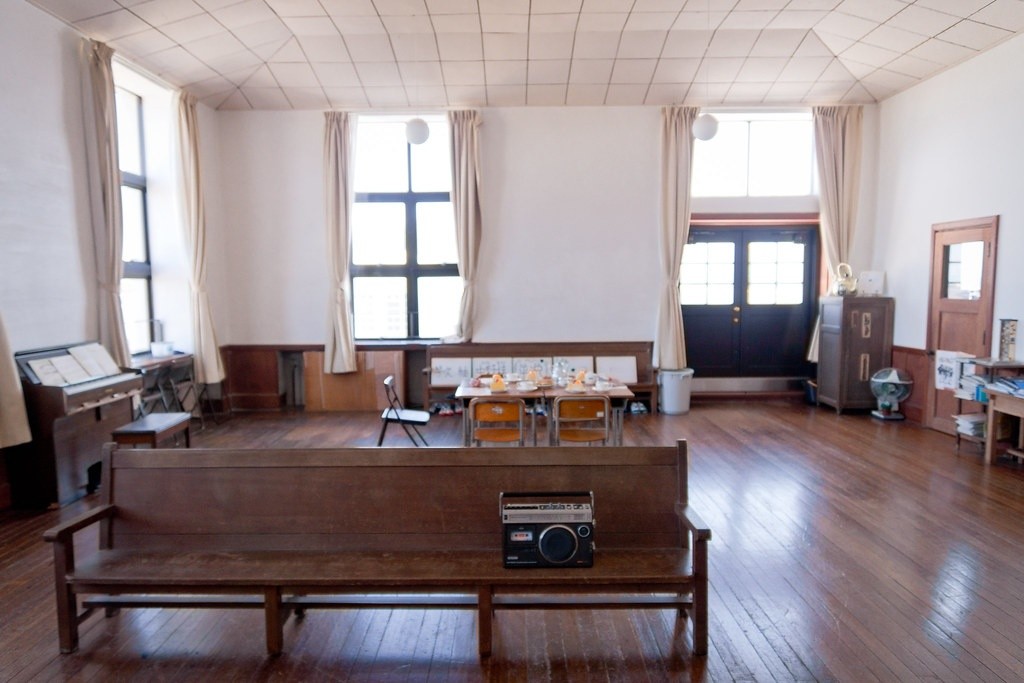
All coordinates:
[422,340,661,413]
[43,440,712,661]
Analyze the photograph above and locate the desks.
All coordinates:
[983,377,1024,469]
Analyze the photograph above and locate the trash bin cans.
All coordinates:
[659,369,694,415]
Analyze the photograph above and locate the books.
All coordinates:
[951,375,1024,436]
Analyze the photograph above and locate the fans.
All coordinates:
[871,367,914,421]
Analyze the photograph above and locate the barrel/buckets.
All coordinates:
[657,368,694,415]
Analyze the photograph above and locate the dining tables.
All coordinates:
[453,380,634,447]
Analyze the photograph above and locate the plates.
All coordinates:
[503,379,522,382]
[564,388,585,393]
[517,386,538,391]
[592,387,611,392]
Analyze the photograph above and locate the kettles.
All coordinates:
[832,263,858,297]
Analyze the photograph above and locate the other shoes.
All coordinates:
[630,402,648,415]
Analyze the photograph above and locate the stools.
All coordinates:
[111,412,193,450]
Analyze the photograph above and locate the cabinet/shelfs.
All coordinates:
[955,356,1024,452]
[816,296,896,414]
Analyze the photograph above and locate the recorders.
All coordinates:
[497,504,596,568]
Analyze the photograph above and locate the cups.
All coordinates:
[585,373,598,382]
[595,381,609,389]
[506,373,518,378]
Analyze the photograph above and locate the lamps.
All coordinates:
[406,13,430,145]
[692,9,718,141]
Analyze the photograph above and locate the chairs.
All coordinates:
[468,373,610,447]
[141,363,183,447]
[377,375,432,447]
[151,356,206,435]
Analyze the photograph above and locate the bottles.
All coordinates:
[538,359,546,378]
[568,368,577,385]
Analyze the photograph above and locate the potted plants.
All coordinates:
[146,318,176,359]
